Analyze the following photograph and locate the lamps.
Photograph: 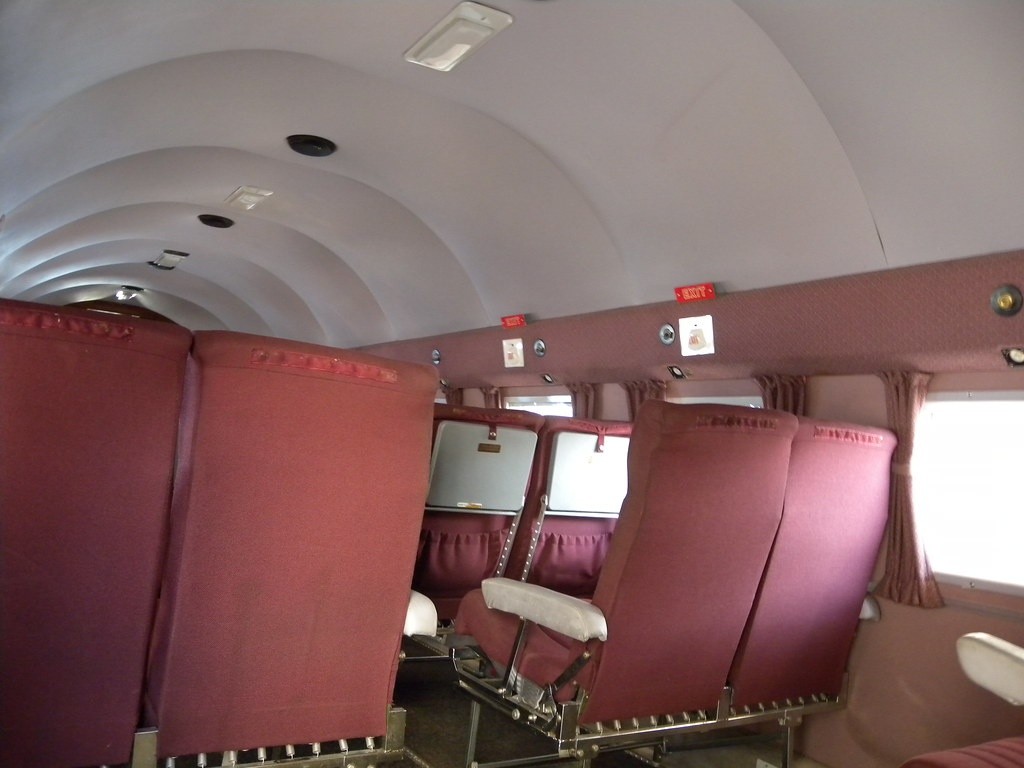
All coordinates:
[113,285,144,302]
[145,246,189,273]
[401,1,514,75]
[223,184,274,212]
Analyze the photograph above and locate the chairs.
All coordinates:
[0,300,1024,768]
